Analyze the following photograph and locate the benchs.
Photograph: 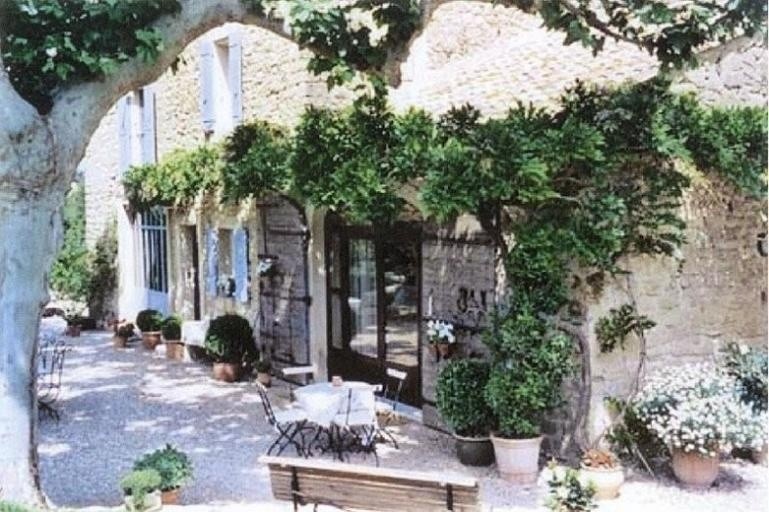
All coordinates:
[261,452,484,511]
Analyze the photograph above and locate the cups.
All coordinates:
[332,375,343,387]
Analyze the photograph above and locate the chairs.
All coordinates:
[252,363,408,466]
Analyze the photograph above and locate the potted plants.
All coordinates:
[118,446,193,512]
[435,310,568,480]
[109,304,259,382]
[579,363,766,500]
